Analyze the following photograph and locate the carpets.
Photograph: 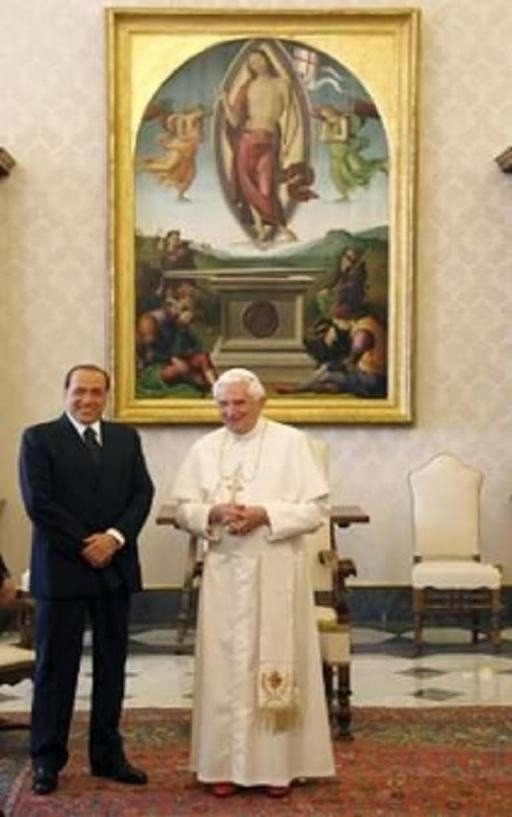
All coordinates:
[1,704,512,817]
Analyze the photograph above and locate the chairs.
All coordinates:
[304,517,356,740]
[407,452,503,653]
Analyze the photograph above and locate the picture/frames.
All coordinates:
[101,6,421,429]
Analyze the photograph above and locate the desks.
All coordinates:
[156,503,373,655]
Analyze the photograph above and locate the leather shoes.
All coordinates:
[213,783,289,796]
[33,769,57,793]
[111,760,145,784]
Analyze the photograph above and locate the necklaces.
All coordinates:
[219,419,268,507]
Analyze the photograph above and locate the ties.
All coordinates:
[85,427,101,467]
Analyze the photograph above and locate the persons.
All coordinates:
[140,103,208,201]
[213,48,298,247]
[318,242,365,371]
[168,364,339,800]
[18,362,156,794]
[274,297,388,399]
[148,228,191,301]
[137,282,220,394]
[314,102,390,202]
